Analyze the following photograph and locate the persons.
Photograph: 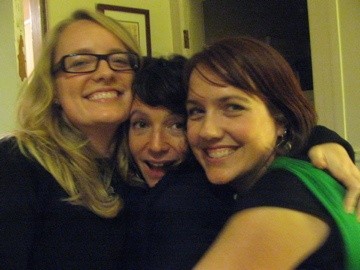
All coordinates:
[184,38,360,270]
[0,9,360,270]
[117,55,189,188]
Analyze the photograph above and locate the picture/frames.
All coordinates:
[96,3,152,58]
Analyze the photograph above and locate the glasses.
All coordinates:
[52,52,139,74]
